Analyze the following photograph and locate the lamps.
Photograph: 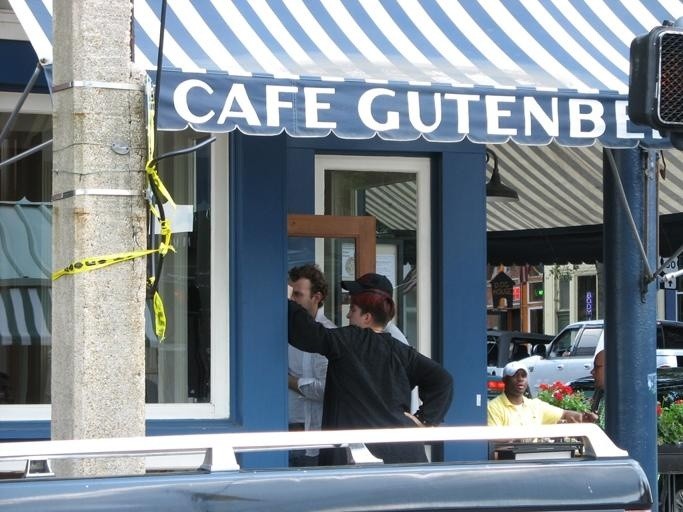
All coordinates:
[484,148,520,202]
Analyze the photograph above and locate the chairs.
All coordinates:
[512,344,546,362]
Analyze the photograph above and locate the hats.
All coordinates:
[502,360,529,379]
[339,272,393,299]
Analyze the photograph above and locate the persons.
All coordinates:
[487,361,583,462]
[287,262,339,467]
[288,287,453,464]
[574,350,607,458]
[341,274,421,414]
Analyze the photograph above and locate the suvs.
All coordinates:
[487,318,682,409]
